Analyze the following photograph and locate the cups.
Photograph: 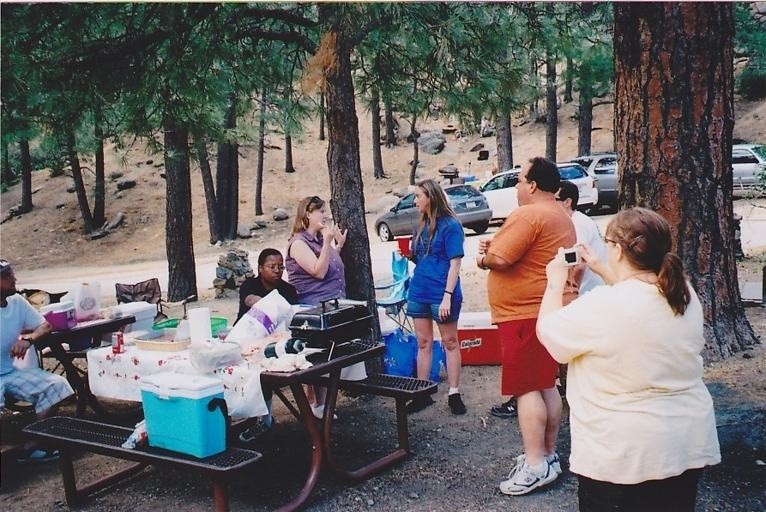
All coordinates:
[398,238,409,256]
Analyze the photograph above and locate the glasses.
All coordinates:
[263,265,285,272]
[307,196,320,211]
[602,235,618,246]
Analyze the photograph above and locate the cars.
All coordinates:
[732,144,766,186]
[374,184,493,242]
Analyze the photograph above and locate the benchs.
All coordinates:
[316,373,437,450]
[43,345,95,358]
[6,397,34,411]
[23,415,261,512]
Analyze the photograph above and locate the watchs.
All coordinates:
[21,335,32,345]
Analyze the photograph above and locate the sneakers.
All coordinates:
[448,392,466,415]
[499,458,558,496]
[512,453,562,474]
[406,395,433,414]
[312,403,338,421]
[491,396,517,416]
[16,447,60,463]
[240,419,270,442]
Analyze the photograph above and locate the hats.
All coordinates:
[0,258,11,273]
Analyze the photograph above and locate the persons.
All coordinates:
[473,157,577,495]
[535,207,721,512]
[0,258,75,462]
[285,196,350,419]
[233,248,298,442]
[490,180,608,418]
[396,178,466,416]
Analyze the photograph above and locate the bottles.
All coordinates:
[263,338,306,358]
[111,331,124,354]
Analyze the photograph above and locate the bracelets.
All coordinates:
[444,290,453,295]
[481,256,485,266]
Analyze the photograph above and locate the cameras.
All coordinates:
[566,247,581,268]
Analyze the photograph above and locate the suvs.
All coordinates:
[571,154,618,215]
[479,163,598,222]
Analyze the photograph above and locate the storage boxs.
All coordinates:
[140,372,228,458]
[39,301,78,331]
[457,311,505,366]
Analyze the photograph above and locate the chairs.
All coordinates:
[116,278,168,324]
[376,251,414,336]
